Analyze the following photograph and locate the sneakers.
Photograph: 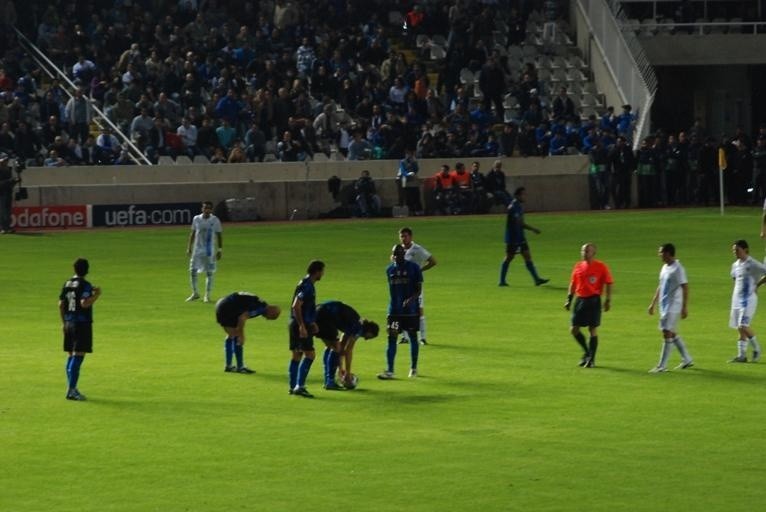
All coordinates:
[203,296,208,302]
[578,360,586,366]
[674,359,693,369]
[648,366,668,373]
[376,371,394,379]
[498,283,508,286]
[223,366,236,372]
[536,279,548,286]
[752,351,760,361]
[583,361,594,368]
[419,339,426,345]
[185,293,199,301]
[65,391,85,400]
[324,383,346,390]
[292,386,313,398]
[726,357,746,363]
[236,368,255,373]
[407,370,416,378]
[288,386,294,393]
[398,338,407,343]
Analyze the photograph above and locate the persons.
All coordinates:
[2,2,640,165]
[1,157,21,233]
[351,169,382,218]
[498,186,550,286]
[648,243,695,374]
[59,258,102,400]
[430,160,514,216]
[564,242,614,368]
[184,200,224,304]
[590,117,764,210]
[397,150,422,215]
[726,240,766,365]
[215,227,437,399]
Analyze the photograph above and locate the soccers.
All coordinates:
[342,372,356,388]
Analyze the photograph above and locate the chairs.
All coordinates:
[264,141,345,162]
[176,156,192,165]
[416,22,607,123]
[158,156,175,165]
[193,155,211,163]
[616,17,744,37]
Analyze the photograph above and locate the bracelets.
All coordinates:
[217,247,223,252]
